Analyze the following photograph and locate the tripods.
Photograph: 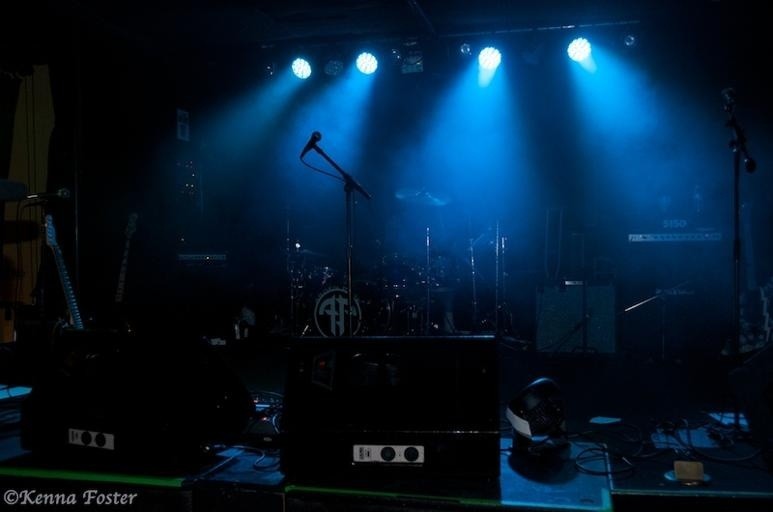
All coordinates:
[399,224,520,338]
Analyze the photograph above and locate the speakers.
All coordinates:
[19,328,216,477]
[279,335,502,501]
[535,283,620,360]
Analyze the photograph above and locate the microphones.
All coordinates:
[25,188,71,200]
[720,86,736,106]
[300,130,321,158]
